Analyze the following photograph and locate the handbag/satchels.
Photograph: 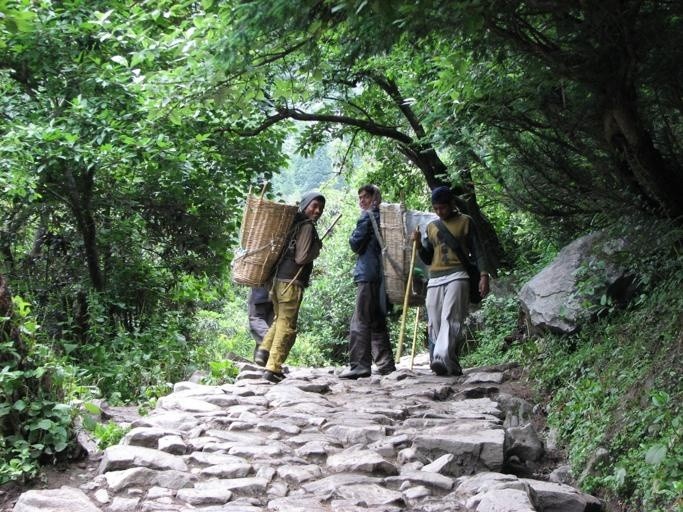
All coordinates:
[469,266,489,304]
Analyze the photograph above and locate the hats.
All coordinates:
[432,186,453,203]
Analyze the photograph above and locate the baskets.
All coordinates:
[379,201,430,306]
[233,192,299,288]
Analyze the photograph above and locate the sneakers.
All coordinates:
[254,350,285,383]
[430,358,463,376]
[339,367,396,379]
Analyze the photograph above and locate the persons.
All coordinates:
[247,287,275,360]
[337,184,396,380]
[254,190,326,385]
[411,186,489,377]
